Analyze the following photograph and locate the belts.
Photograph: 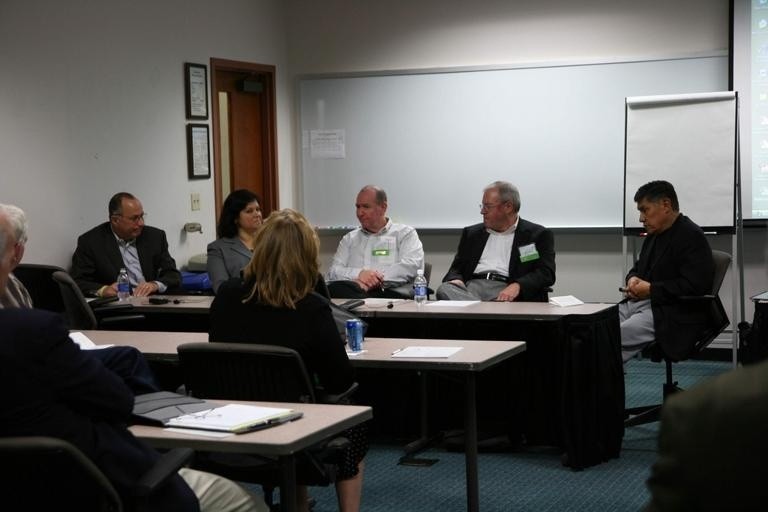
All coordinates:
[471,272,510,285]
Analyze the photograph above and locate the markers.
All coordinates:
[639,233,648,236]
[703,231,717,235]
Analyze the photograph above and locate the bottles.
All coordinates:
[117,268,130,304]
[413,269,428,307]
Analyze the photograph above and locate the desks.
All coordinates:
[68,295,627,512]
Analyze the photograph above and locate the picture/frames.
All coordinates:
[185,122,211,181]
[183,61,209,121]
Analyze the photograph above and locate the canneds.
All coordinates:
[346,319,364,355]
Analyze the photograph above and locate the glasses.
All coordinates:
[121,213,148,223]
[479,202,504,212]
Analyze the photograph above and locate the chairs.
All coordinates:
[51,270,146,332]
[0,434,126,512]
[12,263,146,332]
[619,247,733,429]
[420,261,436,302]
[735,300,767,368]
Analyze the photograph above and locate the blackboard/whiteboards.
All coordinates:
[292,54,731,237]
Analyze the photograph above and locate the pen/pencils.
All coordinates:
[375,269,385,293]
[618,287,631,292]
[392,347,403,354]
[249,420,275,428]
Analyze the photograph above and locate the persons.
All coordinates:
[325,183,425,296]
[434,180,558,301]
[1,202,157,393]
[71,191,182,299]
[209,208,368,511]
[0,207,277,512]
[618,179,714,362]
[205,188,263,296]
